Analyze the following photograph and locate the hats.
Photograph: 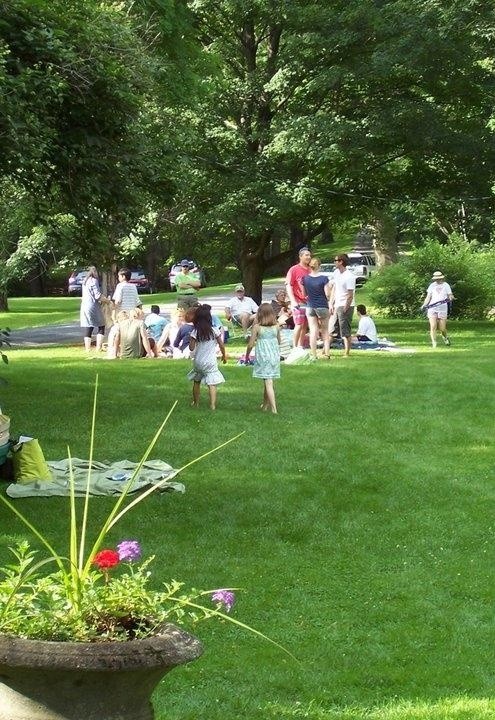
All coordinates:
[431,271,445,280]
[181,259,189,266]
[235,285,245,293]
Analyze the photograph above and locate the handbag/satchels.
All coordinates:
[12,438,52,485]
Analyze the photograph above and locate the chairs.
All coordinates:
[225,307,256,338]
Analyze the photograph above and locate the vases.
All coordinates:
[0,625,204,720]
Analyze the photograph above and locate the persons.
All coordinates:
[108,305,197,360]
[326,253,356,356]
[245,303,282,414]
[300,256,331,359]
[202,304,225,342]
[350,305,378,343]
[111,268,142,315]
[174,259,201,311]
[187,307,227,410]
[227,285,259,334]
[80,266,110,353]
[286,247,313,358]
[271,289,295,329]
[420,271,455,349]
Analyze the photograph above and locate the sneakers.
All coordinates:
[319,355,332,360]
[442,337,450,346]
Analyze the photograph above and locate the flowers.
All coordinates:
[0,372,307,667]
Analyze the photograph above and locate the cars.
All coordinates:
[124,272,159,295]
[344,254,375,286]
[67,269,101,295]
[168,261,208,292]
[318,264,339,281]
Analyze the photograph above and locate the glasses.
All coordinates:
[333,259,341,262]
[183,265,189,268]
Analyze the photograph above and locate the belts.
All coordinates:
[178,293,194,296]
